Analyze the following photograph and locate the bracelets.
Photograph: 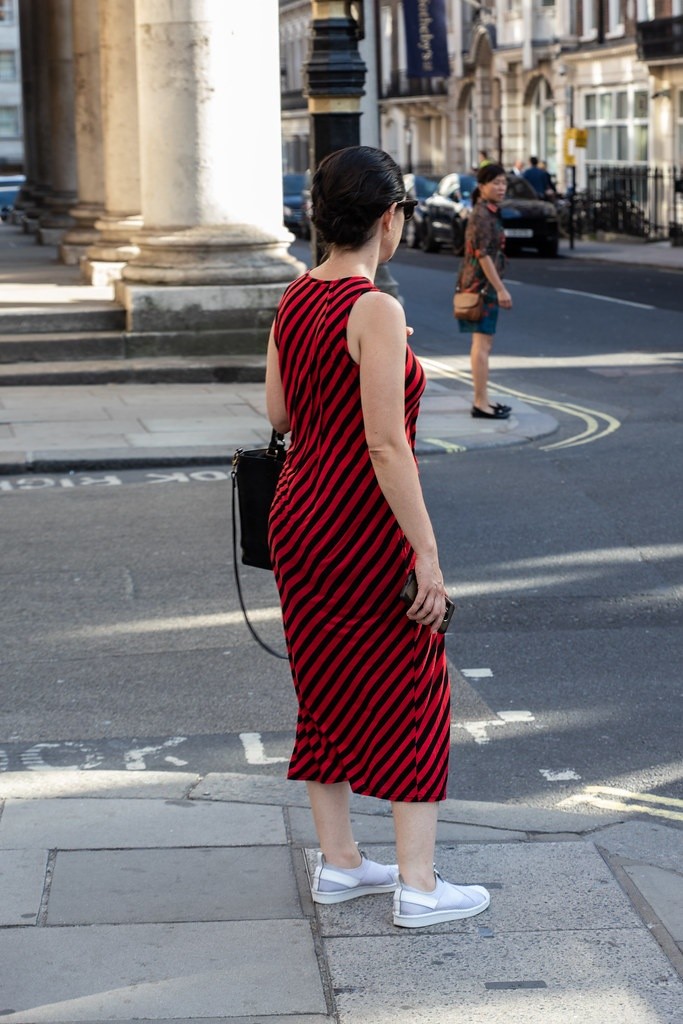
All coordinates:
[496,287,506,291]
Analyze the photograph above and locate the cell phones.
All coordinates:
[400,569,455,633]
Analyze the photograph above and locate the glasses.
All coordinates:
[397,192,418,221]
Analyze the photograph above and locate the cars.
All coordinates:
[413,173,560,259]
[282,171,311,242]
[396,171,444,249]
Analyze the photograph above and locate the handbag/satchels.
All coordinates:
[453,291,484,321]
[231,430,284,570]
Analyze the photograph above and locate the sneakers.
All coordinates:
[393,864,491,929]
[312,841,392,904]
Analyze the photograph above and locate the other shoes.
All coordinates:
[471,405,510,420]
[496,402,512,411]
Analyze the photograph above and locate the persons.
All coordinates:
[512,157,556,201]
[478,149,493,169]
[265,146,493,926]
[456,164,513,419]
